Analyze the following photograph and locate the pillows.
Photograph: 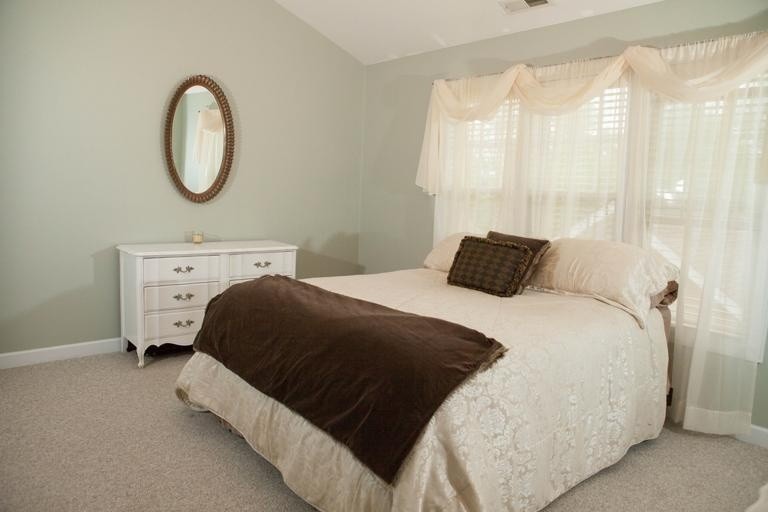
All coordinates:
[422,229,669,330]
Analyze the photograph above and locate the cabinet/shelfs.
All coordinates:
[114,239,299,368]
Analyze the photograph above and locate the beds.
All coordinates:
[215,268,672,511]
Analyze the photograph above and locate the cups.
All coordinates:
[193,231,203,245]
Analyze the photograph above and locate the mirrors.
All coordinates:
[164,75,235,204]
